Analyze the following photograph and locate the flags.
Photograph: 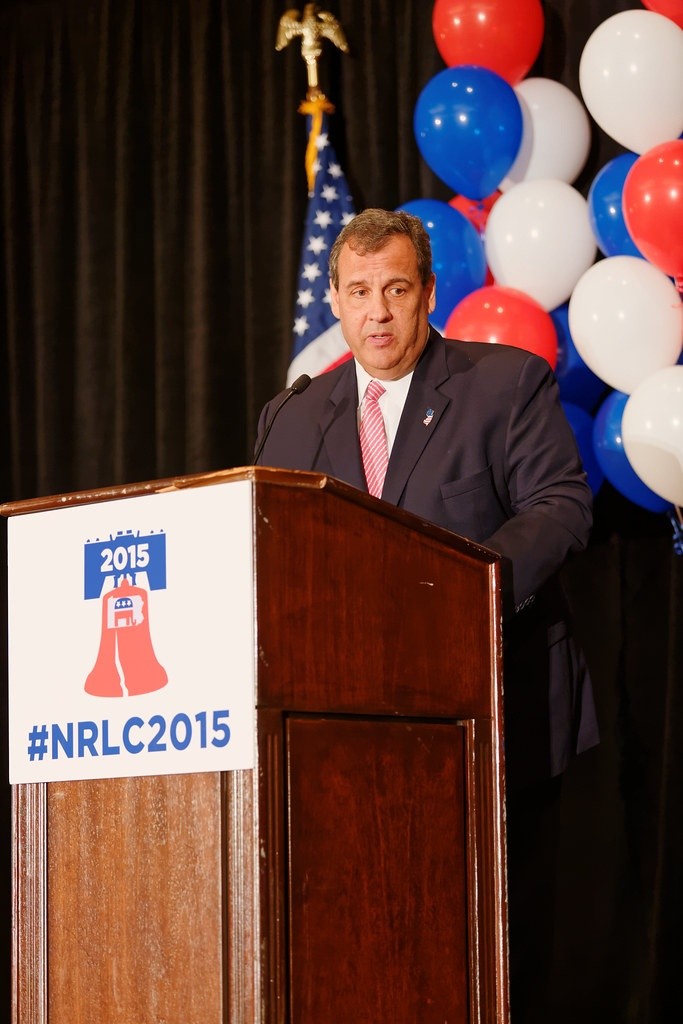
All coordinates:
[286,108,360,392]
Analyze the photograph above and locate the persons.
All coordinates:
[256,208,600,1024]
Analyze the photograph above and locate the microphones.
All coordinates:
[252,374,312,467]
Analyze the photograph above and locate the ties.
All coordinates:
[359,381,389,500]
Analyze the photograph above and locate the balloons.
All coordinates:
[388,0,683,525]
